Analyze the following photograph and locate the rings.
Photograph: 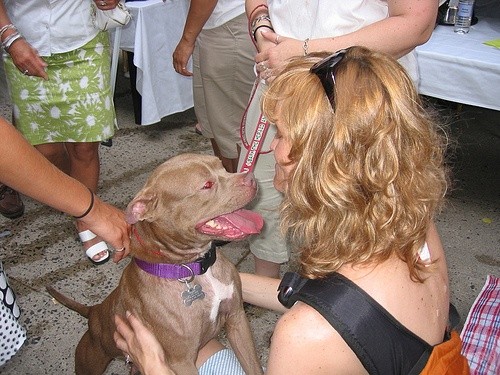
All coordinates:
[101,1,104,5]
[129,237,131,238]
[117,247,125,252]
[182,67,185,69]
[261,62,265,69]
[126,355,129,363]
[277,34,279,41]
[24,70,28,74]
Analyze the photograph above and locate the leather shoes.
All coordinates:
[0,181,24,219]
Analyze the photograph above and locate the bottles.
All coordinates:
[454,0,475,35]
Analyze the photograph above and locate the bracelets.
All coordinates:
[0,23,22,49]
[303,38,308,56]
[73,188,94,218]
[252,16,271,34]
[254,24,275,42]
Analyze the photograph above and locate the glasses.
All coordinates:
[309,45,358,116]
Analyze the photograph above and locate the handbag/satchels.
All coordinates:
[91,0,131,31]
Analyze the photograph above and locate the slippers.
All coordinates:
[76,221,110,265]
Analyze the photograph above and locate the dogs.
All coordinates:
[46,152,265,375]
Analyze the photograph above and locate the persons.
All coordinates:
[0,116,134,366]
[0,0,132,219]
[236,0,439,279]
[115,46,470,375]
[172,0,259,173]
